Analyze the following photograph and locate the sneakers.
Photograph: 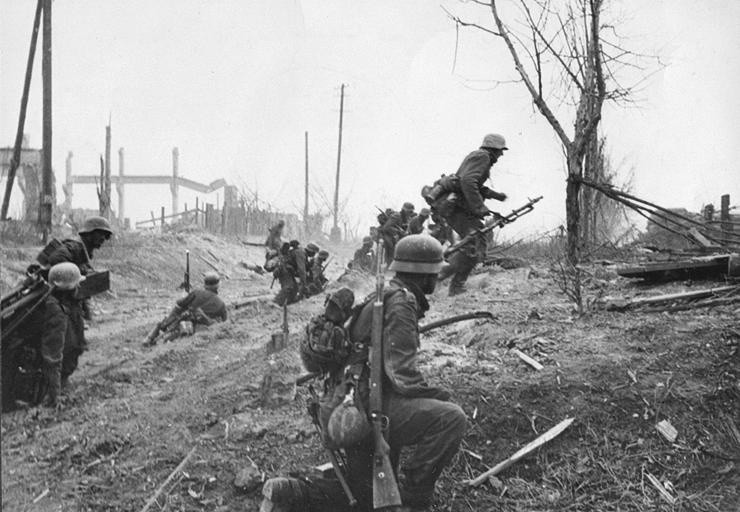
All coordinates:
[258,476,293,511]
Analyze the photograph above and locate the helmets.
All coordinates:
[362,236,373,245]
[203,272,221,289]
[77,215,114,234]
[305,243,320,253]
[401,202,415,211]
[389,232,450,274]
[47,262,87,292]
[419,208,430,217]
[318,250,329,259]
[480,133,510,151]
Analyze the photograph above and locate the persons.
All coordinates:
[144,273,227,347]
[268,241,319,308]
[259,233,469,512]
[269,219,285,249]
[338,202,455,281]
[431,133,509,295]
[292,250,329,302]
[0,261,88,413]
[48,215,114,354]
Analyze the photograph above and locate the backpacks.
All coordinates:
[298,288,408,374]
[377,208,399,225]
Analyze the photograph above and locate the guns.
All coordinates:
[367,238,402,508]
[271,256,285,285]
[374,207,408,235]
[314,253,334,284]
[179,250,191,294]
[444,197,543,257]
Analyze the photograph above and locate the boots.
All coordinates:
[142,323,162,347]
[447,264,470,296]
[436,250,473,282]
